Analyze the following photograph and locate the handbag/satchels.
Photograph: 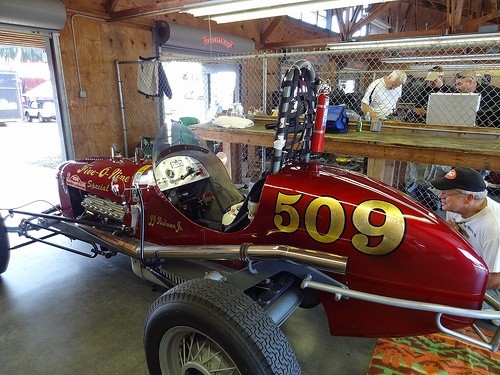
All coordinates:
[326,106,351,133]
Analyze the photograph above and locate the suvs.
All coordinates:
[22,96,57,123]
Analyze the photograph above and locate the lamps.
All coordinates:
[178,0,396,26]
[326,32,500,70]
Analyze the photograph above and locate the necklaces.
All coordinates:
[361,70,407,175]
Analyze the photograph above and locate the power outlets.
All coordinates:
[79,90,86,97]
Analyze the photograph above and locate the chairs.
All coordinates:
[223,159,296,233]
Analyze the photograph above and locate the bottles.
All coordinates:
[356,116,363,132]
[364,111,370,121]
[217,105,254,123]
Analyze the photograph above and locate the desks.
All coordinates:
[187,113,500,190]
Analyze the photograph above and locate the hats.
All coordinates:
[424,71,444,82]
[426,165,485,196]
[455,71,478,80]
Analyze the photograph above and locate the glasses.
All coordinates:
[456,73,474,79]
[440,190,463,198]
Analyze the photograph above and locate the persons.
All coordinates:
[404,66,500,124]
[431,165,500,327]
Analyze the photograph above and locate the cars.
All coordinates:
[1,121,490,375]
